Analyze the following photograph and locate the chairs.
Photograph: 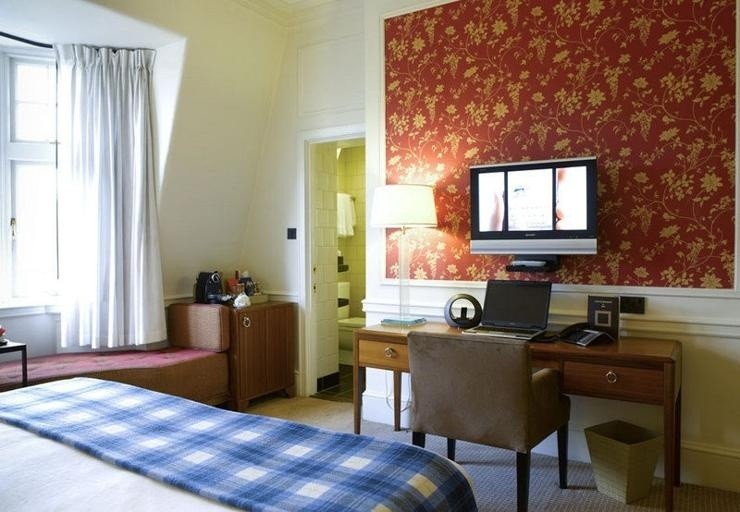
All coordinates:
[408,330,570,512]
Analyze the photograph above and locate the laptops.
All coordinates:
[460,280,553,341]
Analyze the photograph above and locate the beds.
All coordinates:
[0,376,479,511]
[0,303,230,407]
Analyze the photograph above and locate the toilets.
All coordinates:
[336,281,365,351]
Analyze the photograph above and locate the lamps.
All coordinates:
[367,183,439,325]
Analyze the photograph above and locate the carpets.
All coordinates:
[360,418,740,512]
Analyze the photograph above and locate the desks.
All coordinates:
[0,338,29,391]
[353,322,683,512]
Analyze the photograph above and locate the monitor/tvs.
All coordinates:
[468,156,598,256]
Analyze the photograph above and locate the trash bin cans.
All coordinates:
[583,418,664,504]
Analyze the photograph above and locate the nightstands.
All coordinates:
[228,300,297,410]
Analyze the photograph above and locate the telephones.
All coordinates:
[533,322,618,347]
